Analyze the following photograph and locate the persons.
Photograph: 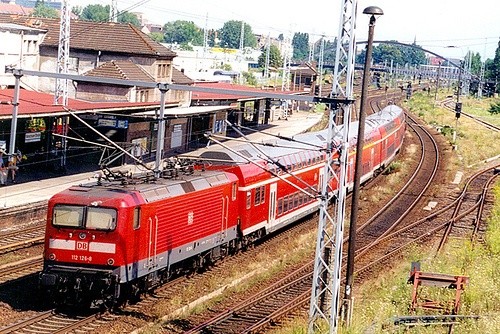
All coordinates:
[0,149,23,184]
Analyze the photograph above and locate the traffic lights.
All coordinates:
[314,85,319,96]
[455,103,463,119]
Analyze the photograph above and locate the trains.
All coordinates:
[41,105,405,313]
